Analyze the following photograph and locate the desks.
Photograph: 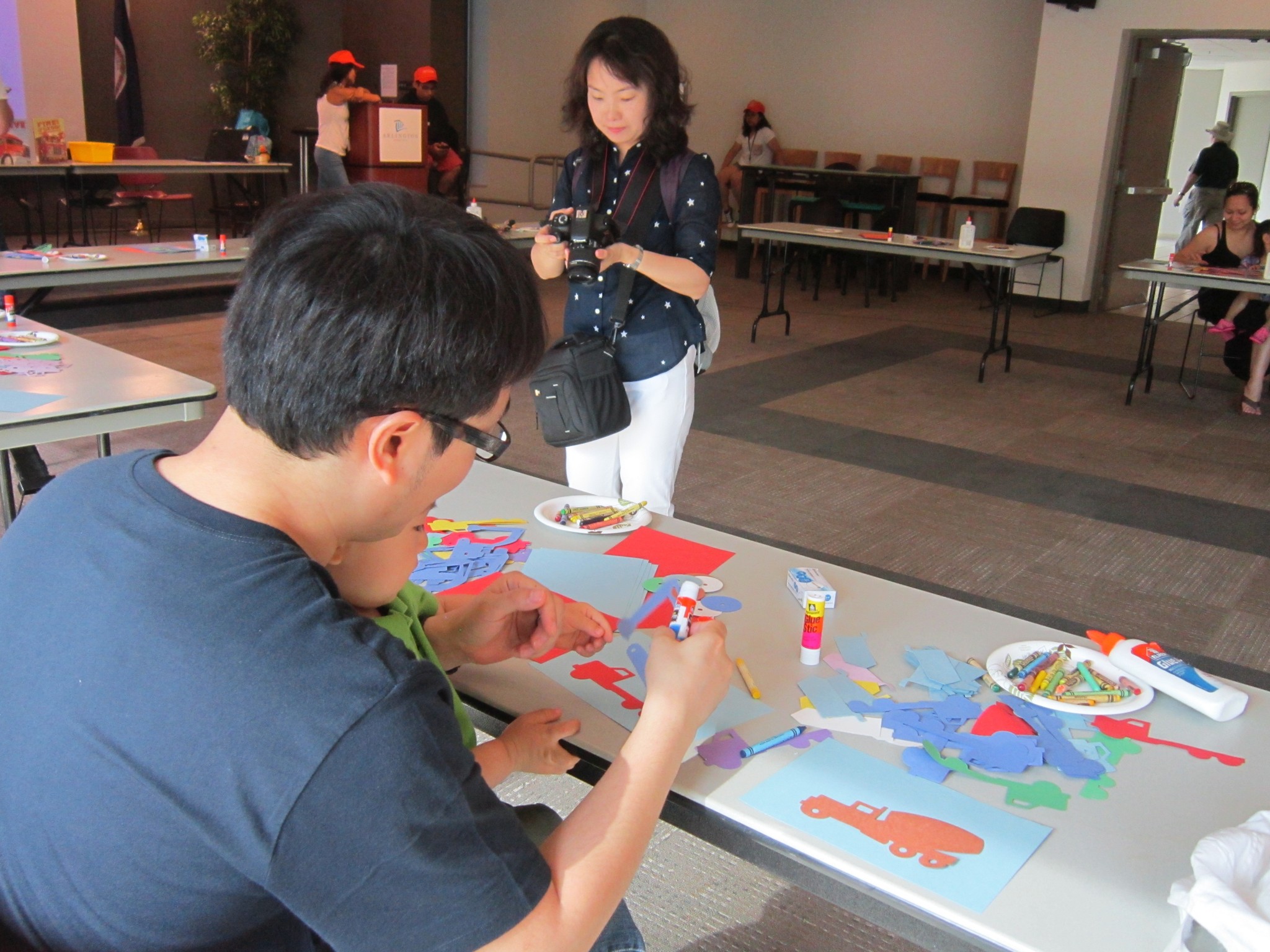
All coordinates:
[0,306,218,526]
[354,450,1270,951]
[1,157,295,252]
[0,237,248,317]
[732,222,1053,383]
[1123,259,1270,414]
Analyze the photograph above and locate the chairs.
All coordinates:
[115,146,206,242]
[1004,205,1067,320]
[734,146,1017,256]
[56,175,108,244]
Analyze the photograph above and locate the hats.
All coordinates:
[328,50,365,69]
[1205,120,1235,141]
[413,66,438,83]
[743,100,765,114]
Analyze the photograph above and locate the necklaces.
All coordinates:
[746,130,757,161]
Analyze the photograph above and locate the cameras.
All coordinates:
[540,205,615,286]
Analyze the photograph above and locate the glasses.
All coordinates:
[356,406,511,463]
[419,85,436,91]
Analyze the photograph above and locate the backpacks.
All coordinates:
[571,145,721,376]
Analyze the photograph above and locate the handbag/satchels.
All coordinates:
[235,108,272,164]
[528,322,632,448]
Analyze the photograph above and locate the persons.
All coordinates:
[0,178,728,952]
[1171,120,1239,267]
[1173,181,1270,417]
[530,16,727,521]
[310,49,381,192]
[1208,219,1270,344]
[395,66,464,203]
[319,497,615,793]
[713,100,782,230]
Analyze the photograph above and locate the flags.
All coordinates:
[109,1,146,154]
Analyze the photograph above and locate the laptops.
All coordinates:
[183,128,259,161]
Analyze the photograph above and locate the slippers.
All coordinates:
[1237,395,1262,417]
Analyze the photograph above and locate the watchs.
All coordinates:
[1178,191,1185,196]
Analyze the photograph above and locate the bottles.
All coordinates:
[1087,630,1249,722]
[958,216,976,250]
[467,197,483,220]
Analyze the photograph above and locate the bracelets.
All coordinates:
[621,243,644,271]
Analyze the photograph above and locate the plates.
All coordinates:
[0,331,59,346]
[534,496,652,535]
[812,228,842,233]
[59,253,108,262]
[983,245,1015,252]
[986,641,1154,715]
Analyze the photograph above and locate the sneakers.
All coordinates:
[724,207,735,228]
[1249,326,1270,345]
[1208,318,1236,333]
